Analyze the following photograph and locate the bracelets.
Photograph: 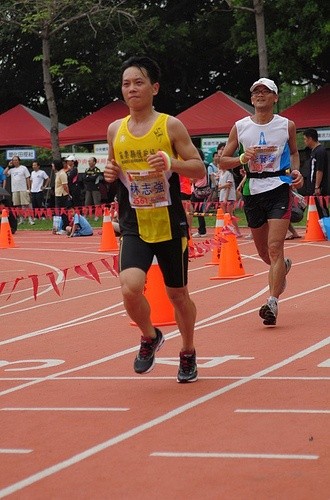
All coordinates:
[239,153,247,164]
[8,165,11,169]
[290,169,300,174]
[157,151,171,171]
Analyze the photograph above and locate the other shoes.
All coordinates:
[34,218,38,221]
[244,235,253,240]
[56,230,66,234]
[84,215,88,220]
[94,216,99,221]
[235,232,242,238]
[192,232,208,238]
[42,217,45,221]
[17,221,25,225]
[28,220,35,225]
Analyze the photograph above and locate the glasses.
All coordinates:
[251,89,275,95]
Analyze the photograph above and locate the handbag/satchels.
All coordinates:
[194,185,212,199]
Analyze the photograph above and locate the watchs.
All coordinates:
[314,185,320,189]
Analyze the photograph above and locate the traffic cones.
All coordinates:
[187,226,204,259]
[300,196,327,242]
[208,211,254,280]
[204,208,225,266]
[0,209,20,248]
[96,207,121,251]
[127,254,178,327]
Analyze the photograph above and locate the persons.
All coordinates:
[104,56,206,383]
[220,78,304,325]
[0,156,120,237]
[285,206,304,239]
[186,143,253,239]
[303,129,330,209]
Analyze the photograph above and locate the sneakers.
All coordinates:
[259,298,278,326]
[176,348,199,382]
[134,327,165,375]
[279,256,292,296]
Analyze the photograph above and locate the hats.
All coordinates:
[250,78,278,95]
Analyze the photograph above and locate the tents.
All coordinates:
[173,91,255,137]
[279,83,330,129]
[0,105,68,152]
[59,100,131,153]
